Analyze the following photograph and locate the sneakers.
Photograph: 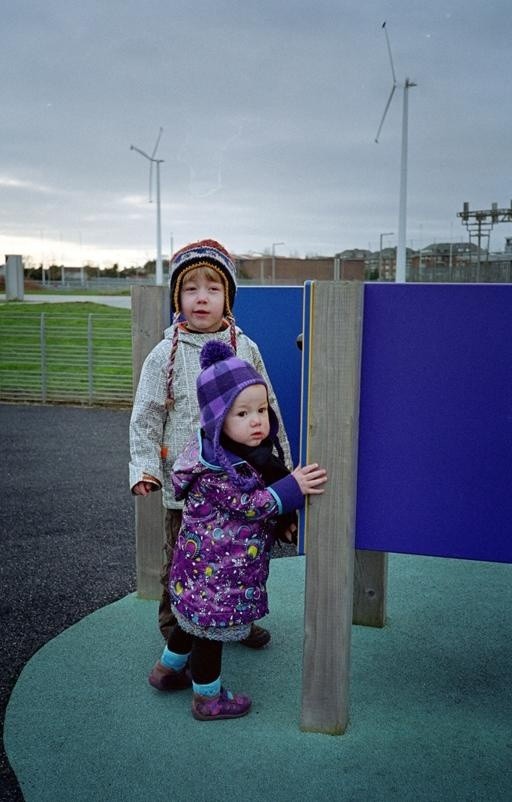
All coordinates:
[191,686,252,720]
[149,660,192,691]
[238,623,271,648]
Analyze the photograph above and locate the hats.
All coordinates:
[165,239,237,411]
[196,341,285,494]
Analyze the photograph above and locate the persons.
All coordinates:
[127,239,293,648]
[147,338,328,721]
